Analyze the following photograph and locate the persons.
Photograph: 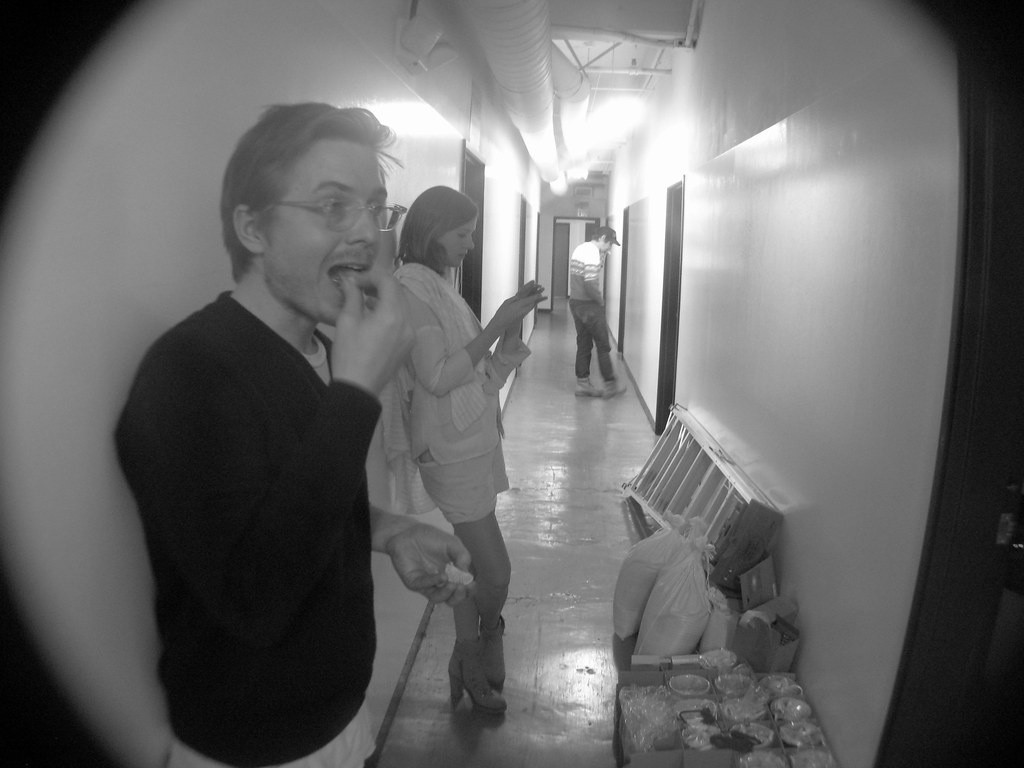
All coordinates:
[111,103,480,768]
[379,185,548,715]
[569,227,627,399]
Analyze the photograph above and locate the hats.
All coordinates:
[597,227,621,246]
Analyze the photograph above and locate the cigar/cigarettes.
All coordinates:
[607,250,611,255]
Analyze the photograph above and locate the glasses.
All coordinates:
[272,197,401,232]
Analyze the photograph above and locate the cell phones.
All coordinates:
[527,286,545,299]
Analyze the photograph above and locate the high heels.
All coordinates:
[481,613,505,689]
[447,636,507,714]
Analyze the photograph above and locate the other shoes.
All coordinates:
[604,380,627,398]
[574,379,604,398]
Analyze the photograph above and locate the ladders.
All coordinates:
[623,405,785,590]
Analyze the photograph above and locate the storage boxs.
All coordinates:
[613,669,836,768]
[727,557,802,673]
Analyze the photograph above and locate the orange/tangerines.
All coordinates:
[446,561,474,586]
[336,268,359,284]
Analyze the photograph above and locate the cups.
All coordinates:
[669,674,821,750]
